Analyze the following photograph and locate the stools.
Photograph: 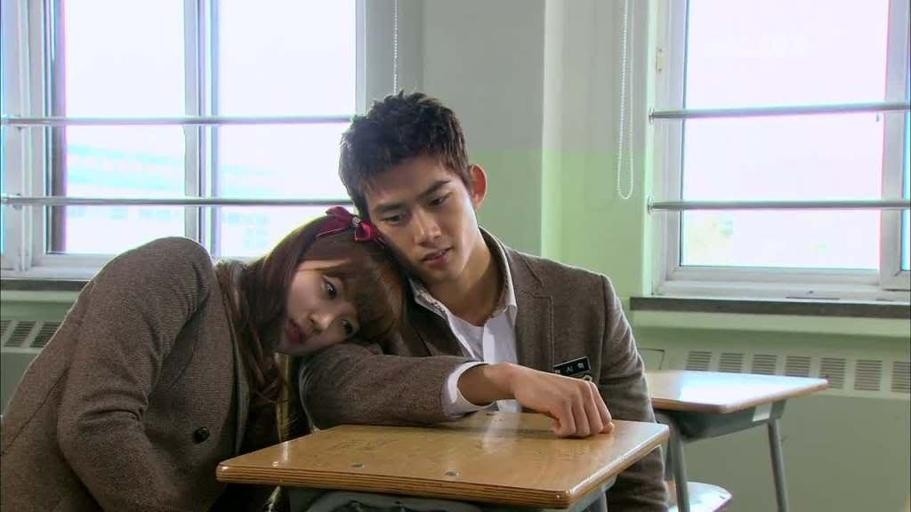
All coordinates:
[665,476,731,512]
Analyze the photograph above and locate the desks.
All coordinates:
[649,372,830,512]
[215,410,669,511]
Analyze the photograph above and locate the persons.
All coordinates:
[1,206,403,512]
[274,87,672,511]
